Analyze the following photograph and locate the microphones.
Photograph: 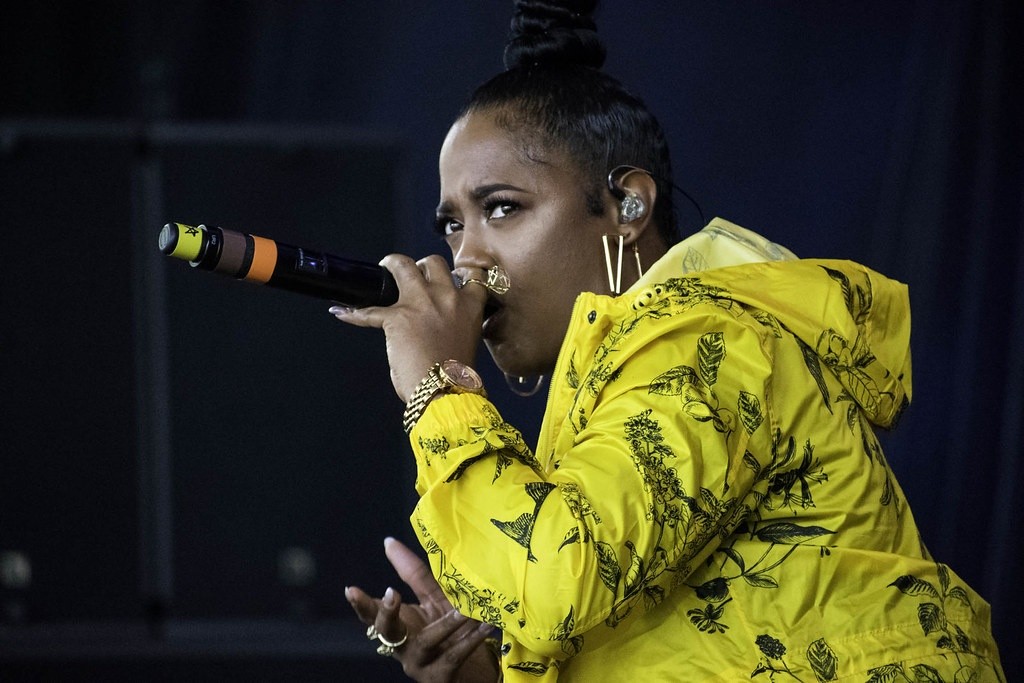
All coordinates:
[157,223,463,309]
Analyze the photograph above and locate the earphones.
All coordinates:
[608,180,645,224]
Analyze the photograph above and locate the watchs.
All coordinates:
[403,359,488,436]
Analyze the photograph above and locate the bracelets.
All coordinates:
[486,645,504,682]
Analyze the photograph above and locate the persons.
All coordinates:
[328,0,1004,683]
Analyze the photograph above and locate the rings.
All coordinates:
[366,623,408,655]
[459,265,511,296]
